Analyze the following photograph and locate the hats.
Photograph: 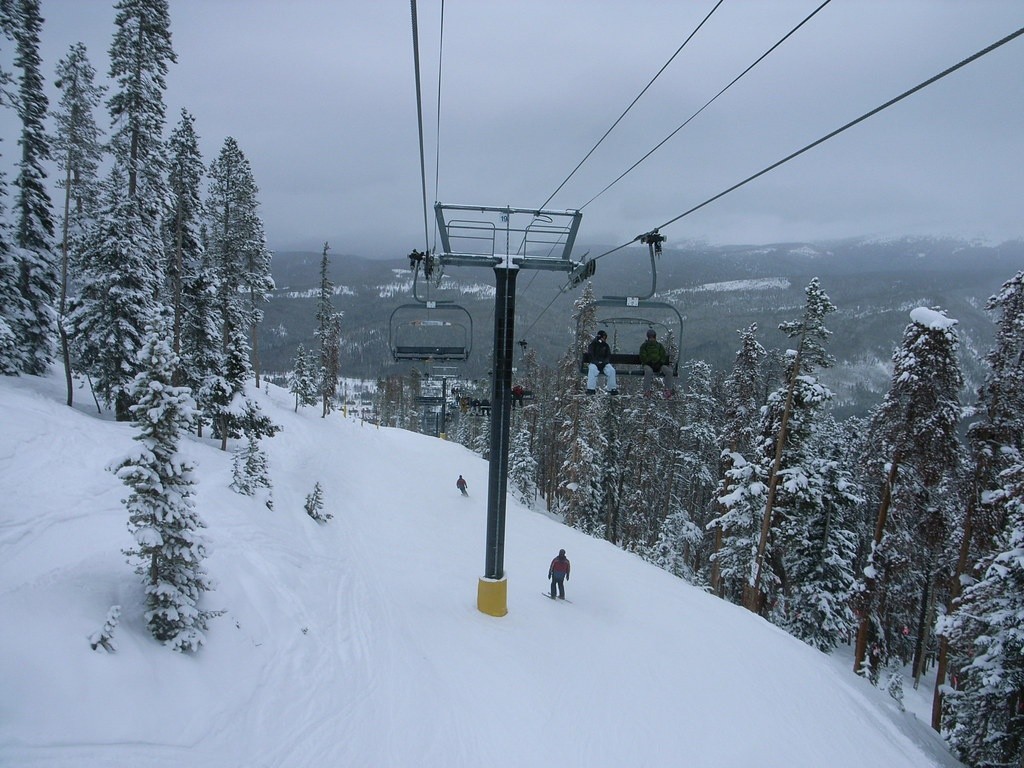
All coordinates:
[647,329,656,337]
[598,330,607,339]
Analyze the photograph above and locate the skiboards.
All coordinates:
[542,592,573,605]
[573,394,632,398]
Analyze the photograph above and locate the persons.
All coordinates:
[586,330,619,396]
[451,388,481,416]
[512,385,524,410]
[548,549,570,600]
[457,475,468,497]
[482,399,491,416]
[639,330,673,400]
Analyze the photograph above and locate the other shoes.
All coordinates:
[551,595,556,600]
[611,389,619,395]
[586,389,595,396]
[643,390,651,399]
[663,390,671,398]
[558,596,565,599]
